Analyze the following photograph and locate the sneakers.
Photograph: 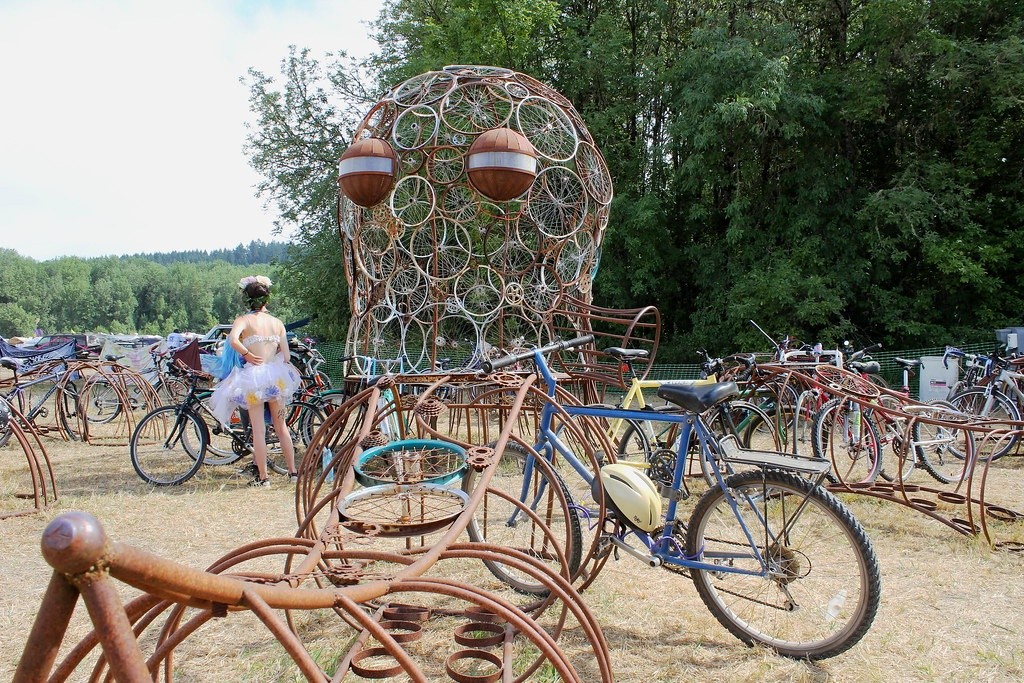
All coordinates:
[288,470,298,484]
[246,477,270,488]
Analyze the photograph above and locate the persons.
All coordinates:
[202,276,299,488]
[167,329,192,378]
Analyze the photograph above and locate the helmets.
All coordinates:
[590,462,661,534]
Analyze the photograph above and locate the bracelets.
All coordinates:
[242,351,249,356]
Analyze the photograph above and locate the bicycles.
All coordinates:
[131,315,406,487]
[0,345,89,449]
[75,345,189,424]
[412,337,502,406]
[592,332,1024,490]
[461,336,880,659]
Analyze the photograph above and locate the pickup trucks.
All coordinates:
[24,335,104,359]
